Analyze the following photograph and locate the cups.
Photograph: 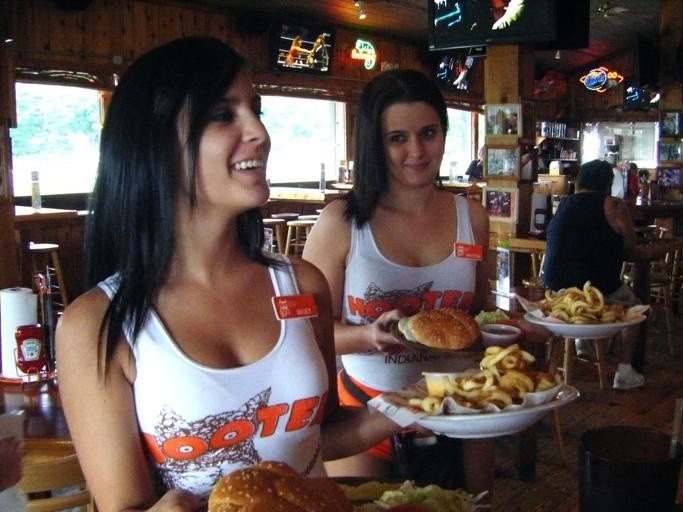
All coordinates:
[13,324,46,373]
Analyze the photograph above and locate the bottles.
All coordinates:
[338,159,347,184]
[30,170,40,215]
[536,121,568,139]
[347,161,353,184]
[532,177,560,195]
[318,162,326,193]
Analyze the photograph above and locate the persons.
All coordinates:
[284,36,311,67]
[54,35,431,511]
[542,160,645,392]
[302,68,495,479]
[307,35,326,68]
[465,144,485,182]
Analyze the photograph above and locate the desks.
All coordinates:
[438,180,475,198]
[15,204,95,283]
[503,234,682,377]
[267,186,352,215]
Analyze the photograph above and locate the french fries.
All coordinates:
[410,344,558,412]
[541,280,631,325]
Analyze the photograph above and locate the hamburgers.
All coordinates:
[207,460,353,512]
[398,306,481,351]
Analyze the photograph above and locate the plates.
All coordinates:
[523,306,648,340]
[414,385,580,440]
[388,316,547,360]
[188,476,486,512]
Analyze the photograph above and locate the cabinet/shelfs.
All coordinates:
[536,121,582,178]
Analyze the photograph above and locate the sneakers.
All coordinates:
[574,337,596,360]
[612,369,645,390]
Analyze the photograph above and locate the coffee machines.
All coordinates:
[601,134,623,166]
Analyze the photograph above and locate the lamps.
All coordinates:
[353,0,367,19]
[626,86,662,104]
[555,50,561,61]
[593,0,624,24]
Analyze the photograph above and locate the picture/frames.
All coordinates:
[481,187,519,224]
[658,109,683,139]
[656,167,683,193]
[484,104,523,138]
[659,137,683,168]
[521,143,539,183]
[484,144,520,181]
[520,101,535,145]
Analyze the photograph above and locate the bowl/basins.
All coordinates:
[476,322,523,349]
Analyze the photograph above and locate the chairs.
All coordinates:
[21,437,98,512]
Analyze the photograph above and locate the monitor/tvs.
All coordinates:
[623,77,660,110]
[432,50,475,93]
[427,0,590,51]
[268,20,334,74]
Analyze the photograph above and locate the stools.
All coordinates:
[546,335,610,390]
[26,242,70,323]
[623,247,679,355]
[262,213,320,258]
